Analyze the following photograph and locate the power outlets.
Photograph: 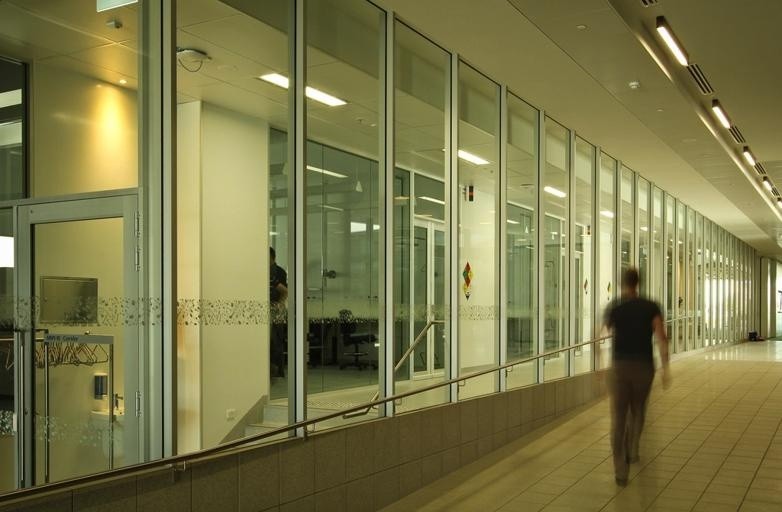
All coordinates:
[742,144,758,168]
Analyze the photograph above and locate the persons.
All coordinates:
[593,269,674,490]
[269,246,288,378]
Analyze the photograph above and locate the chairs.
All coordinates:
[337,308,377,372]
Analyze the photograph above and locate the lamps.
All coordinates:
[712,96,730,130]
[762,175,774,195]
[655,12,691,69]
